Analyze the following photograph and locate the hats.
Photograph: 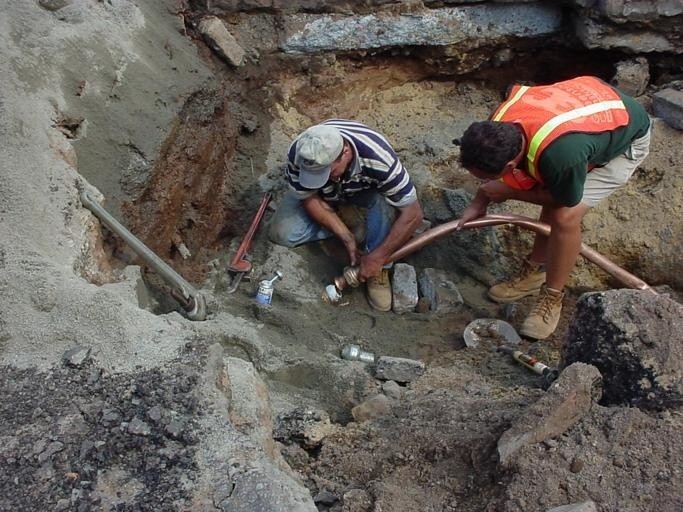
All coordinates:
[294,125,343,189]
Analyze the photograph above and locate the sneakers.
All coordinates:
[486,255,566,340]
[337,203,368,244]
[366,269,392,312]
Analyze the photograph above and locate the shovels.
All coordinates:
[463,318,560,384]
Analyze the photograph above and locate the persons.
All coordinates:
[453,73,650,342]
[265,116,425,313]
